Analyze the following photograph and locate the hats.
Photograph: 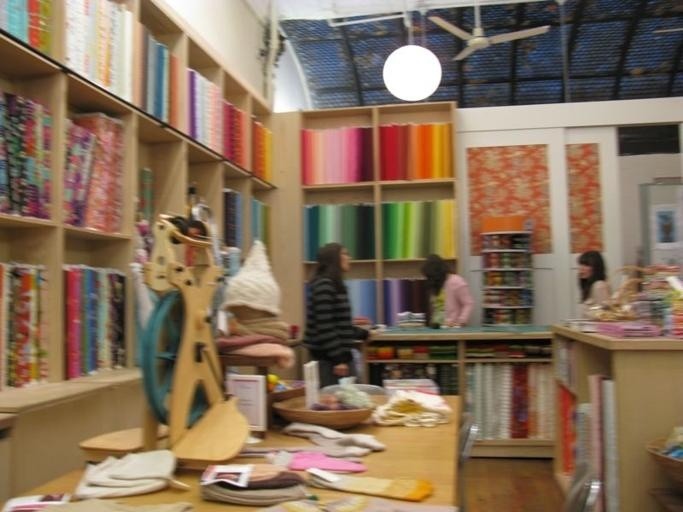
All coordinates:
[71,448,190,502]
[200,463,318,506]
[219,239,280,315]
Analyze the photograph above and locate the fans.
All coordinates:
[424,1,551,64]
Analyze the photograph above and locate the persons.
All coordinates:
[571,250,613,313]
[420,252,474,328]
[299,240,373,389]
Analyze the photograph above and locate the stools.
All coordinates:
[79,415,176,466]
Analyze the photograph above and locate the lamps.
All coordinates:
[380,0,444,103]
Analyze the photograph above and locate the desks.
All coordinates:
[0,385,466,511]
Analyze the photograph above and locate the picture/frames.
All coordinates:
[651,201,683,251]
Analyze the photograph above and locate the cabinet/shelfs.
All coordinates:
[0,1,279,507]
[554,319,682,511]
[360,324,555,460]
[283,102,466,332]
[480,231,538,326]
